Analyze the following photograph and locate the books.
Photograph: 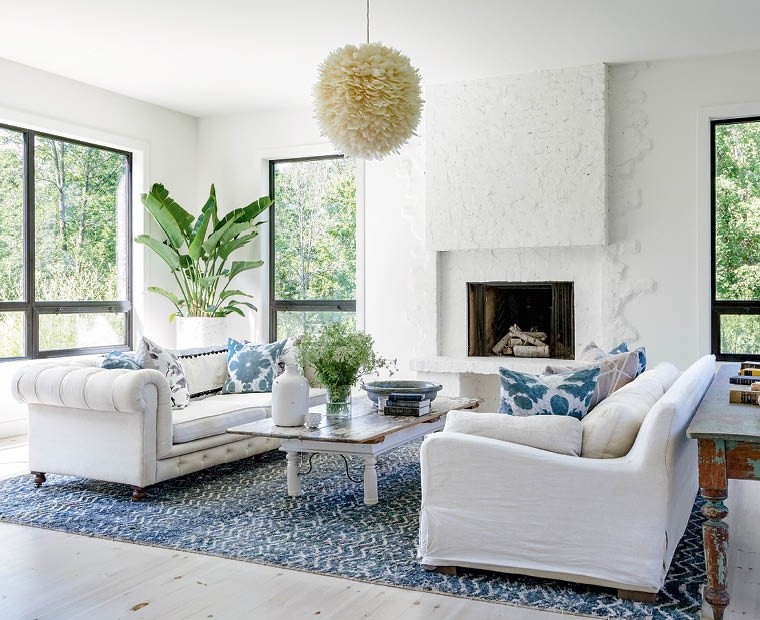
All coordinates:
[729,360,760,404]
[386,399,430,408]
[384,405,430,417]
[388,393,425,401]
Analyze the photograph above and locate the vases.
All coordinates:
[322,382,353,415]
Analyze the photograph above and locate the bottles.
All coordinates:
[271,364,310,427]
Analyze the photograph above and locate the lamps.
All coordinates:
[312,1,426,162]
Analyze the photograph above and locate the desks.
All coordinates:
[686,364,760,620]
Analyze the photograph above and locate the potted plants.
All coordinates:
[135,181,275,348]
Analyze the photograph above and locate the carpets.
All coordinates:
[0,405,720,620]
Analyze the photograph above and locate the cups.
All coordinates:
[305,413,322,428]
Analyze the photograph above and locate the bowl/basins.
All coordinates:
[362,380,443,408]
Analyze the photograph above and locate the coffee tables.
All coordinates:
[227,396,484,504]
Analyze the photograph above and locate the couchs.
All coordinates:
[417,356,721,603]
[11,344,328,499]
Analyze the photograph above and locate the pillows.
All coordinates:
[444,340,678,460]
[133,336,193,408]
[99,351,144,370]
[218,337,289,396]
[244,336,305,380]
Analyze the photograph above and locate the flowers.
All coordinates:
[296,326,382,392]
